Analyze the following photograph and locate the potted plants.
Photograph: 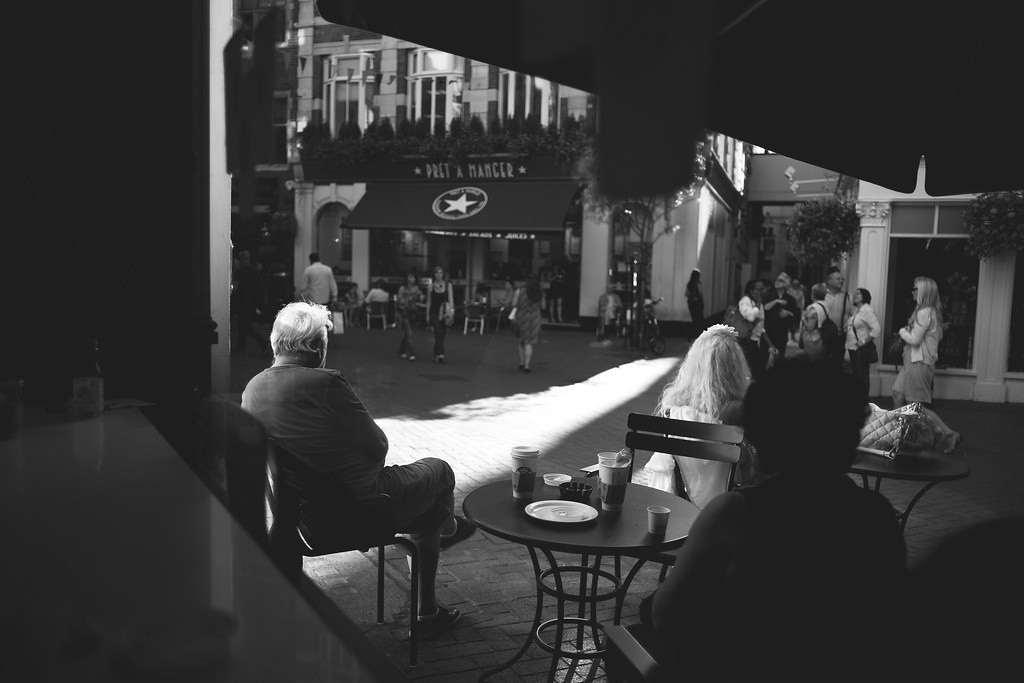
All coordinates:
[781,196,857,270]
[965,192,1024,262]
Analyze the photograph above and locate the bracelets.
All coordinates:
[861,339,867,345]
[801,316,805,321]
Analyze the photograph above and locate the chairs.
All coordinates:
[625,410,744,577]
[263,441,419,672]
[463,304,488,335]
[494,291,518,336]
[362,301,389,331]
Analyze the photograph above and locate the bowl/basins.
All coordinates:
[559,482,593,503]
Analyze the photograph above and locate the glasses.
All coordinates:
[912,286,918,293]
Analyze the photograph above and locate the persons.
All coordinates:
[398,273,423,360]
[512,272,542,374]
[633,323,751,509]
[240,301,477,639]
[548,264,569,322]
[738,272,851,353]
[364,271,397,329]
[685,267,705,336]
[470,280,514,334]
[426,264,455,363]
[643,355,912,683]
[293,253,338,305]
[596,283,622,341]
[341,283,363,326]
[891,275,944,408]
[846,288,882,403]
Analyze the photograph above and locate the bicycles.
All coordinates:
[637,297,667,356]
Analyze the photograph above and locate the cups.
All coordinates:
[511,446,541,499]
[597,451,631,512]
[647,506,671,535]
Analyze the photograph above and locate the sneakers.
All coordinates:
[440,514,477,551]
[409,606,462,641]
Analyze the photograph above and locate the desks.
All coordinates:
[849,454,970,540]
[461,478,703,683]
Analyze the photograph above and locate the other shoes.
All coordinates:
[409,356,415,360]
[399,354,407,358]
[439,354,444,362]
[433,356,439,362]
[524,369,531,373]
[518,365,524,370]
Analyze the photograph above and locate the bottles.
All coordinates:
[0,376,24,440]
[72,336,104,419]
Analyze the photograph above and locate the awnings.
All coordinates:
[310,0,1023,202]
[336,176,582,232]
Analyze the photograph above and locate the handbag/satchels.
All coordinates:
[332,311,344,334]
[508,308,518,324]
[723,295,762,345]
[814,302,839,346]
[887,337,906,359]
[847,342,879,364]
[856,402,962,460]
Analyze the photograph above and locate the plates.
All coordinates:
[524,500,598,523]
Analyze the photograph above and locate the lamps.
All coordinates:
[784,166,841,194]
